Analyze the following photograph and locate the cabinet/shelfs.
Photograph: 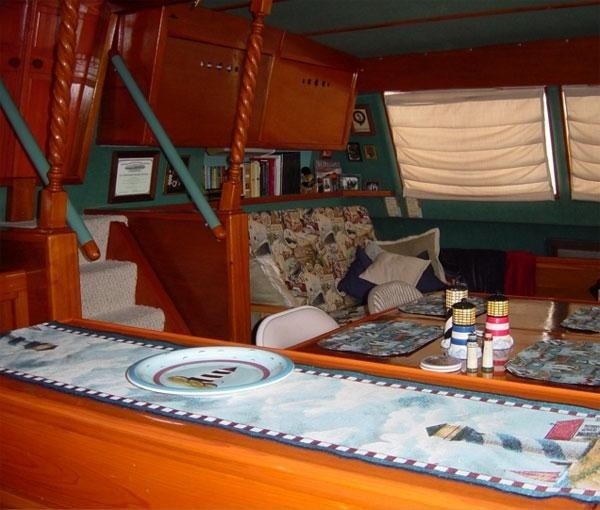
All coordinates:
[96,4,357,151]
[0,0,117,187]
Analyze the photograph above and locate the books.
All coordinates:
[204,147,303,199]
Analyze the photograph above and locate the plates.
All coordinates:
[126,345,296,394]
[420,355,461,372]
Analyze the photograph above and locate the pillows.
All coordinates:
[338,228,453,301]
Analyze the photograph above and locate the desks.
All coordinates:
[283,292,600,411]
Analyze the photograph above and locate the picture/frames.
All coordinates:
[108,150,161,204]
[163,155,190,196]
[354,103,375,137]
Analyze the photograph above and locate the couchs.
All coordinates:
[247,206,464,332]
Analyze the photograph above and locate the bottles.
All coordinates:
[440,284,511,374]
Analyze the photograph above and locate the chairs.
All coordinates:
[504,249,600,303]
[368,280,421,314]
[255,307,338,349]
